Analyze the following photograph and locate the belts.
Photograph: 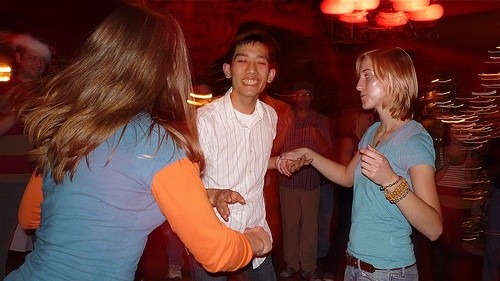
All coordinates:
[344,254,418,273]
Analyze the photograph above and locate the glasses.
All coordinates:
[298,93,310,97]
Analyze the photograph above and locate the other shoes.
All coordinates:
[280,266,300,278]
[301,270,321,281]
[323,273,335,281]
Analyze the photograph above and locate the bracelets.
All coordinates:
[380,176,410,205]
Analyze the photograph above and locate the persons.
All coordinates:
[278,31,374,281]
[192,29,314,281]
[277,47,444,281]
[0,4,273,281]
[0,41,49,279]
